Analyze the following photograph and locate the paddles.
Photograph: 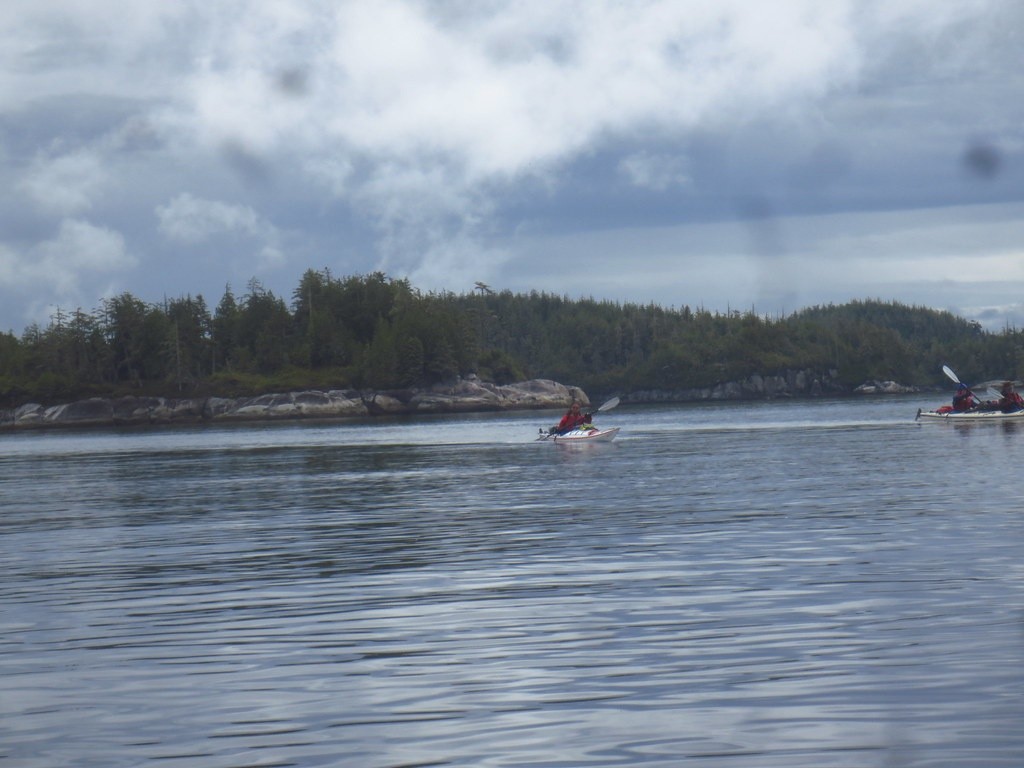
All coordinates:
[537,396,621,441]
[986,386,1024,409]
[942,364,982,403]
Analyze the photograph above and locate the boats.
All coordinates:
[538,425,621,442]
[915,405,1024,419]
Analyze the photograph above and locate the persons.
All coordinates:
[950,383,978,414]
[998,382,1024,411]
[558,402,591,435]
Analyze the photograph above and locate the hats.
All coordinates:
[1002,382,1015,390]
[957,384,968,391]
